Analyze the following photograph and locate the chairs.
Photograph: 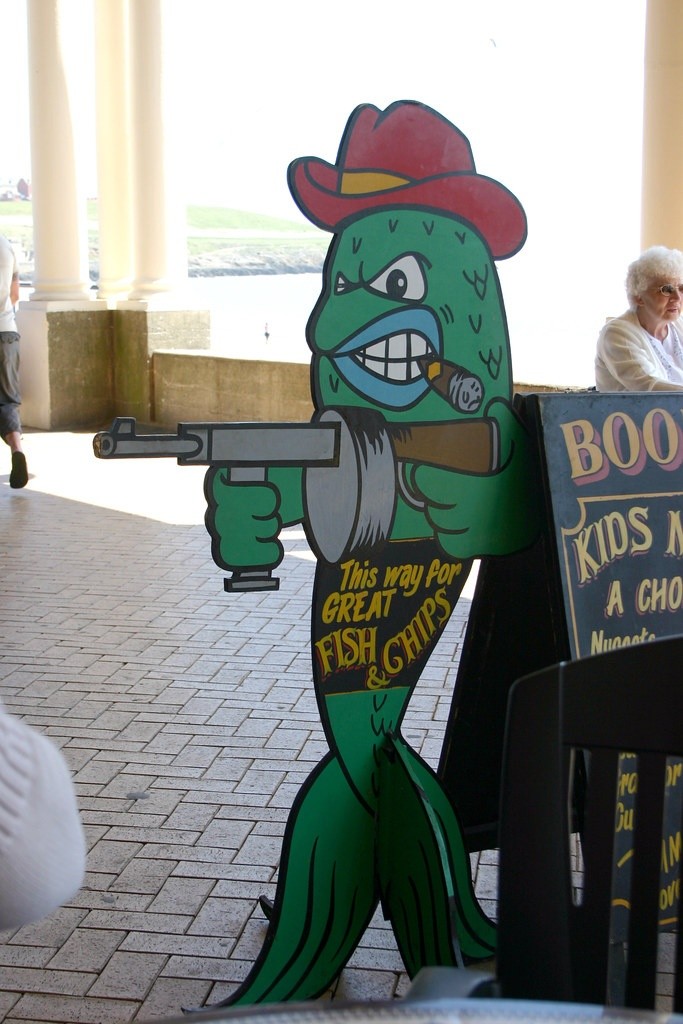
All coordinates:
[404,634,683,1017]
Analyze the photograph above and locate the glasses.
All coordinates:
[646,282,683,298]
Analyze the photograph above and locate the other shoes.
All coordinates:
[9,452,28,489]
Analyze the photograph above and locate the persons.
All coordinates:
[594,247,683,392]
[0,235,29,489]
